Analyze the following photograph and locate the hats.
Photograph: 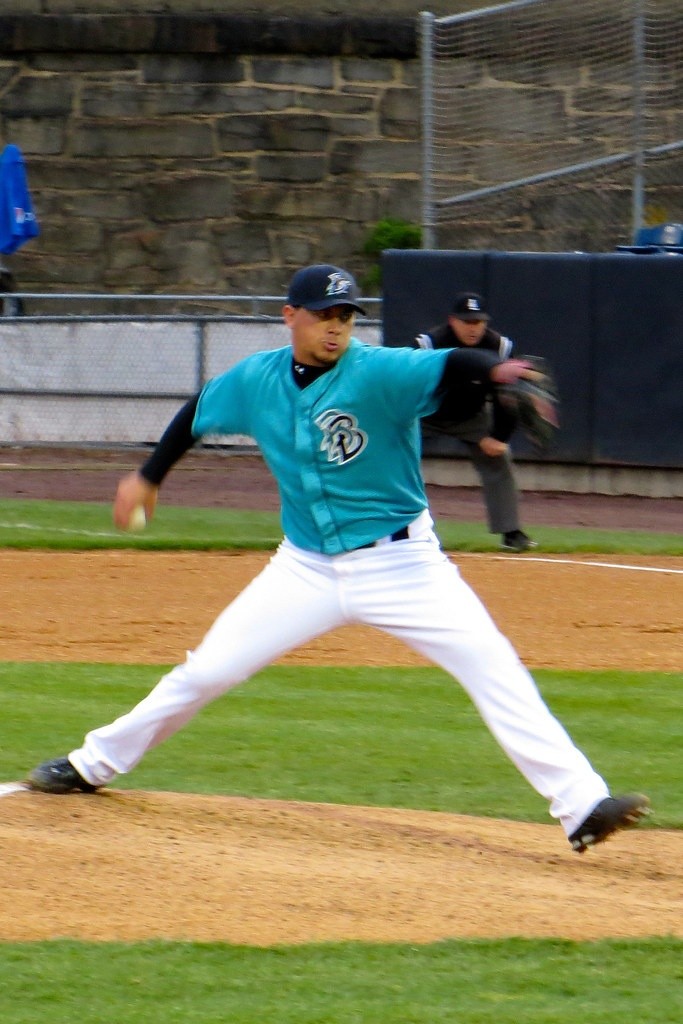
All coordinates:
[449,292,492,321]
[287,262,369,317]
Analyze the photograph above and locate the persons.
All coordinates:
[402,292,539,555]
[20,263,656,856]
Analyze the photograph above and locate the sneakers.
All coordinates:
[23,756,106,795]
[564,791,654,855]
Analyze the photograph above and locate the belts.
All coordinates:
[356,524,411,550]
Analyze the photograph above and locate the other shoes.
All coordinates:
[501,529,539,551]
